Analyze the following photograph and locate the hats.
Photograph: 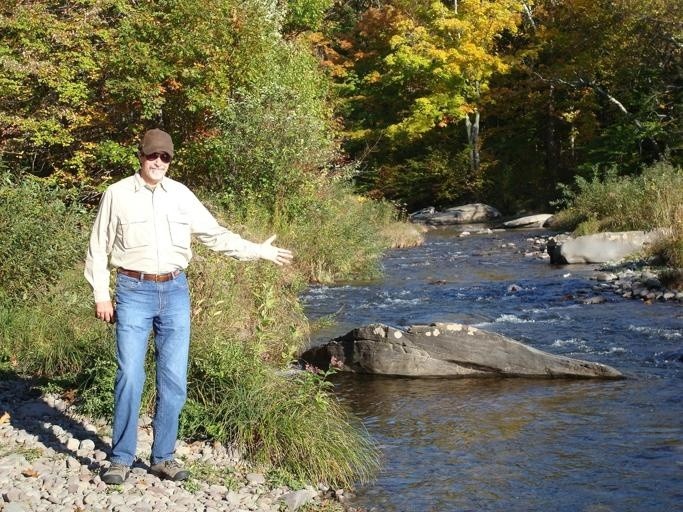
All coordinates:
[139,128,174,159]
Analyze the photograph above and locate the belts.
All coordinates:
[118,268,180,282]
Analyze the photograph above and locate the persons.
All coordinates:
[82,128,293,484]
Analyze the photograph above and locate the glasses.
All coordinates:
[146,152,171,163]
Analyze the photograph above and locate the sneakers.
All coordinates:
[102,462,130,485]
[149,460,189,482]
